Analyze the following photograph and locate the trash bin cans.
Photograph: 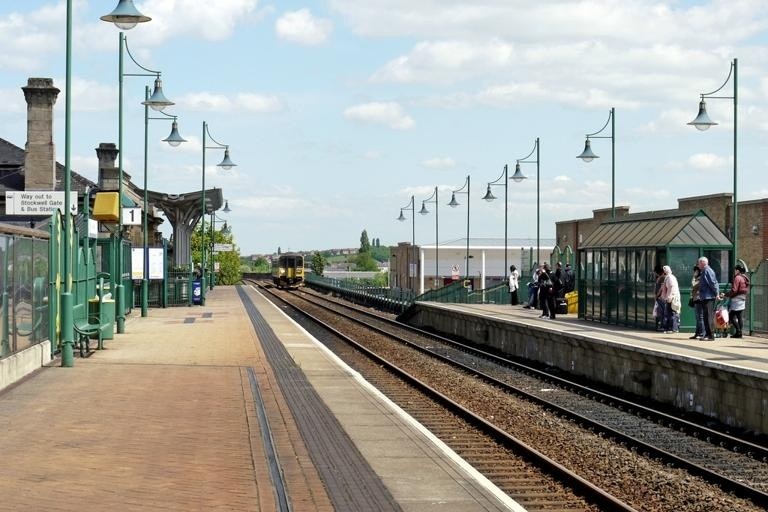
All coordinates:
[88,293,115,350]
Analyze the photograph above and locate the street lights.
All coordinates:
[57,1,152,364]
[141,86,188,319]
[508,138,541,272]
[113,32,176,333]
[212,211,231,287]
[685,57,741,283]
[396,173,471,293]
[481,164,509,283]
[198,120,238,306]
[210,186,232,290]
[576,107,615,219]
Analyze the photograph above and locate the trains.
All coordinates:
[271,253,306,290]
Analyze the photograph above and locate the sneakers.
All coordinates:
[523,305,538,309]
[690,334,714,340]
[730,329,742,338]
[540,315,555,320]
[656,327,679,334]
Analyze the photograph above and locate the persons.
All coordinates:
[654,264,667,330]
[507,261,576,319]
[698,255,722,342]
[689,264,706,340]
[660,265,682,334]
[720,264,750,339]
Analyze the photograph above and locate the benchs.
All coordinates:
[72,303,111,358]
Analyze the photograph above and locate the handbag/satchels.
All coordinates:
[688,298,694,307]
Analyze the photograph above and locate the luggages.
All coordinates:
[556,297,568,313]
